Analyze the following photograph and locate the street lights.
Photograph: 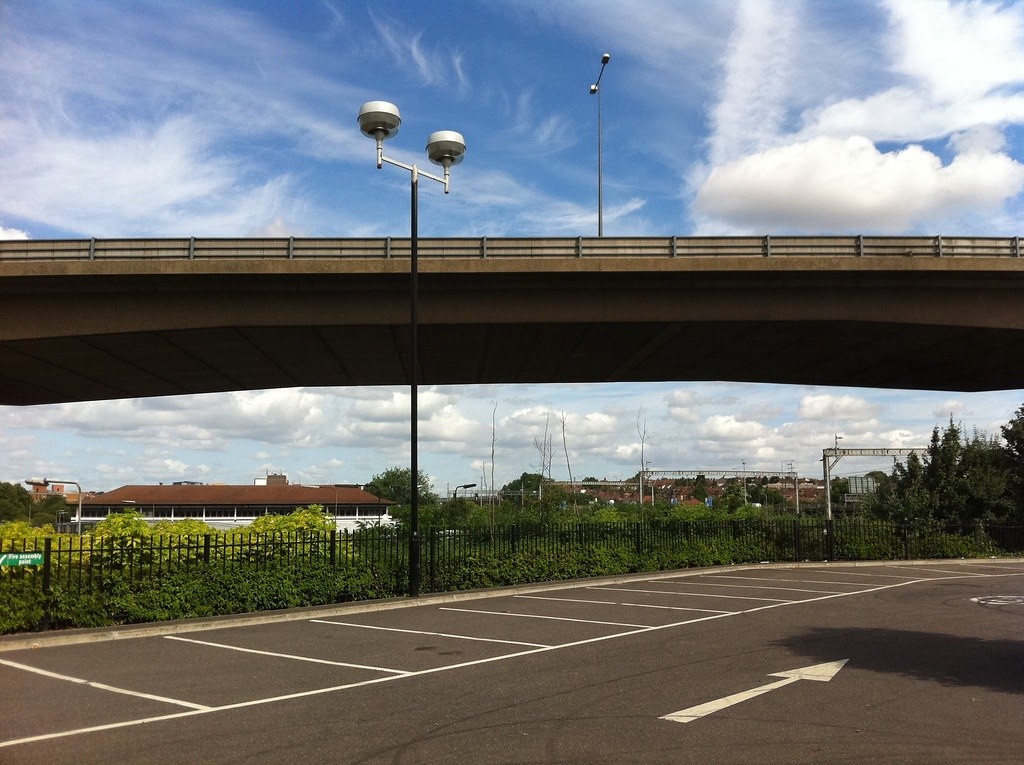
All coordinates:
[589,54,610,235]
[357,101,466,598]
[453,483,477,500]
[25,477,82,536]
